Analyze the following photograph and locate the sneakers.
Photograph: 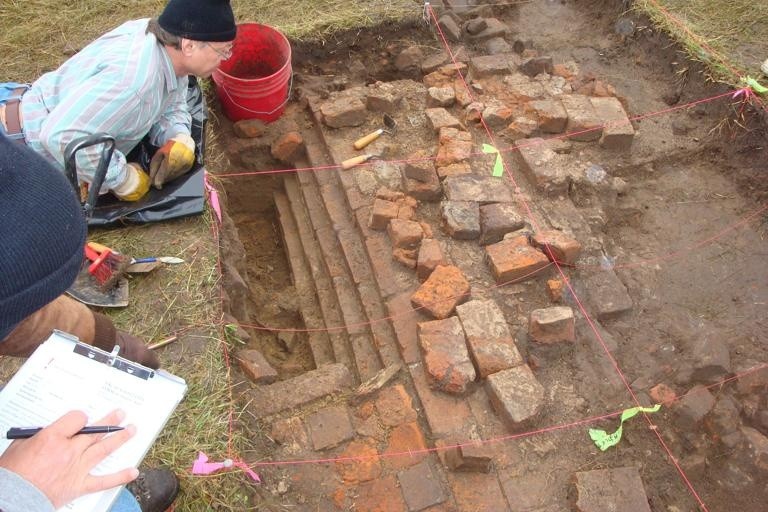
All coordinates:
[126,466,178,512]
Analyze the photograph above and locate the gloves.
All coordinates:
[115,330,161,371]
[149,140,196,190]
[112,161,151,203]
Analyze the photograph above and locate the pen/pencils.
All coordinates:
[2,426,125,439]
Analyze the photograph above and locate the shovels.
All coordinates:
[341,147,390,170]
[353,113,395,150]
[88,242,161,272]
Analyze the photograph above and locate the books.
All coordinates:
[0,326,189,512]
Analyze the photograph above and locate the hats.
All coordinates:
[0,119,91,341]
[157,0,236,42]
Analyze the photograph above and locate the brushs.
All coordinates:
[85,243,133,292]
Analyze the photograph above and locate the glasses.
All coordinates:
[208,42,233,63]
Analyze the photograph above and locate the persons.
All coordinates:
[0,131,183,512]
[1,1,237,204]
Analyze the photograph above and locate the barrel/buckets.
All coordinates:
[213,22,294,124]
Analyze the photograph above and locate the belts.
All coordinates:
[6,81,32,144]
